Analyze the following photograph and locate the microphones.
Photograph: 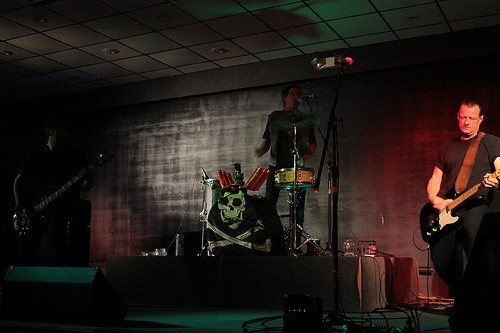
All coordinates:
[298,95,314,102]
[335,57,354,66]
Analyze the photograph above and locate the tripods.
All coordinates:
[283,101,328,256]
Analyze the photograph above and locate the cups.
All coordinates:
[155,248,167,256]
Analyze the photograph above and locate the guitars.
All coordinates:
[8,152,116,241]
[419,169,500,245]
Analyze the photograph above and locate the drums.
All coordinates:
[199,178,222,223]
[274,167,316,191]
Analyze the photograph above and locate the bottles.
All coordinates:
[344,239,358,256]
[175,233,185,256]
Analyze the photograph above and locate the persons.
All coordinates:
[14,113,93,267]
[426,95,500,315]
[253,84,317,256]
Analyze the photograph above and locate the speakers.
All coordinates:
[448,211,500,333]
[2,265,128,327]
